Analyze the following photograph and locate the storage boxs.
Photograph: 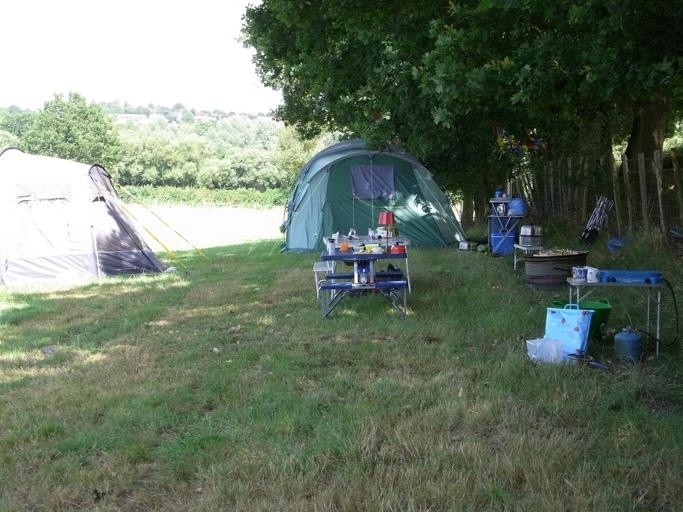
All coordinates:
[490,235,513,256]
[487,217,514,235]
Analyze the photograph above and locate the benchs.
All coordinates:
[312,233,412,317]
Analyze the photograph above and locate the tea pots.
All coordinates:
[390,241,406,253]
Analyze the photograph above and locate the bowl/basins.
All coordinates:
[519,235,542,246]
[352,244,381,253]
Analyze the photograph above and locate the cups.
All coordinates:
[494,192,501,198]
[572,265,600,283]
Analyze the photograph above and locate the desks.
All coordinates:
[565,277,663,358]
[512,245,541,270]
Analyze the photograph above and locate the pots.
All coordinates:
[567,349,607,370]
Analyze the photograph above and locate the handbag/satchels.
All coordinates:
[545,304,595,356]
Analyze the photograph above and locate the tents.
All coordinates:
[0,147,178,292]
[280,139,468,253]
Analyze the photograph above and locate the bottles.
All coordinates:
[386,264,399,272]
[496,203,505,216]
[359,268,367,284]
[326,238,335,253]
[346,228,359,240]
[367,226,385,240]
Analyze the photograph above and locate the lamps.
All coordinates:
[379,211,397,249]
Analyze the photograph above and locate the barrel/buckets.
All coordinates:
[549,297,612,361]
